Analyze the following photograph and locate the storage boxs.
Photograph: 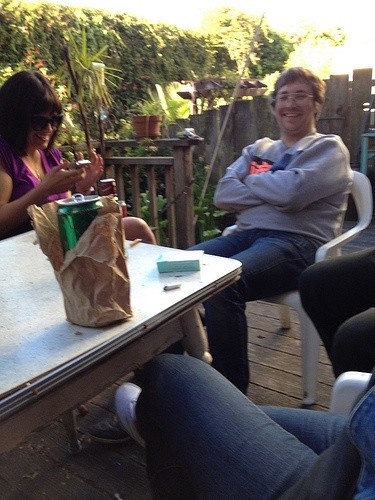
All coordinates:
[156,250,204,273]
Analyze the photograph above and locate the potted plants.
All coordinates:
[131,100,165,139]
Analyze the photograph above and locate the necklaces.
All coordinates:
[26,155,40,179]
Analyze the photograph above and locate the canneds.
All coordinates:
[56,193,104,261]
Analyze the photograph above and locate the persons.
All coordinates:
[298,244,375,377]
[0,70,187,356]
[183,66,353,398]
[114,353,375,500]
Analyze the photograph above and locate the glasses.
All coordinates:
[29,114,64,131]
[271,92,316,106]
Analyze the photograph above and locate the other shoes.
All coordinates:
[113,382,147,449]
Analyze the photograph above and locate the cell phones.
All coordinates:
[70,160,91,169]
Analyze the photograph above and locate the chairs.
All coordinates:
[220,170,373,405]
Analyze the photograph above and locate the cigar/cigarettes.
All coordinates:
[163,283,182,290]
[130,238,142,247]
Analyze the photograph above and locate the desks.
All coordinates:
[0,228,243,457]
